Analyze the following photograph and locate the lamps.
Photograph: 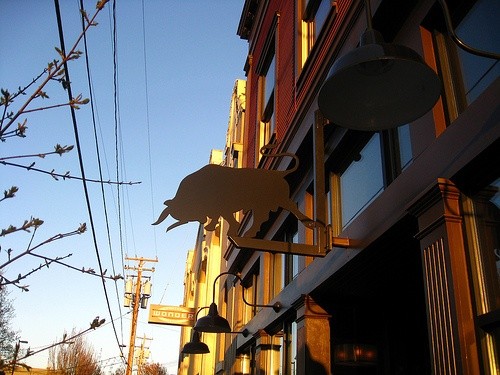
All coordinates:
[318,0,500,132]
[181,306,209,355]
[193,271,282,333]
